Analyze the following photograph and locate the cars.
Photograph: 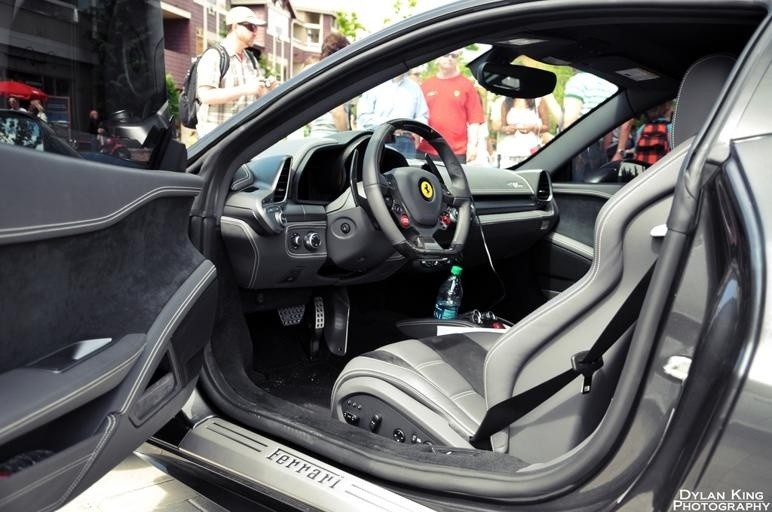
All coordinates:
[0,0,771,512]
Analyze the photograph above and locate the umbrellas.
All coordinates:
[1,80,50,102]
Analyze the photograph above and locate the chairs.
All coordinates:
[329,53,745,467]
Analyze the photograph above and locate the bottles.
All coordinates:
[432,264,466,321]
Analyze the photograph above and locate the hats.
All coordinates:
[226,6,266,27]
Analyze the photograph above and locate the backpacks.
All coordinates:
[179,43,228,130]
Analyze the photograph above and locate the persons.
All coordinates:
[417,49,485,165]
[306,31,350,138]
[8,94,29,115]
[195,6,279,139]
[354,72,430,150]
[466,69,675,185]
[29,98,48,123]
[86,110,107,154]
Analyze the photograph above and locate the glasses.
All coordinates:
[239,24,258,33]
[444,52,458,59]
